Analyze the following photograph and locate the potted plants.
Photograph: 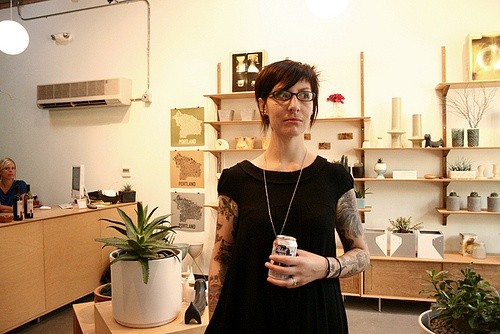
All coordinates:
[388,217,424,258]
[94,283,112,304]
[446,192,500,212]
[118,183,136,203]
[331,155,387,209]
[418,260,500,334]
[446,155,476,178]
[94,200,181,327]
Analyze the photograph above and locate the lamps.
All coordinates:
[0,0,29,55]
[50,32,73,44]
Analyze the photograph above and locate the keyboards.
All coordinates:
[57,204,73,209]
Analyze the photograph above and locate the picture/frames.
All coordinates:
[468,32,500,82]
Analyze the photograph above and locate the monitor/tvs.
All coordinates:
[71,165,90,207]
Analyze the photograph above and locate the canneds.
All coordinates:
[268,235,297,280]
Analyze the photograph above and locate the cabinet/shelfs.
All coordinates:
[334,248,500,313]
[0,199,141,334]
[197,80,500,226]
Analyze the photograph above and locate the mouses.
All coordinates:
[40,205,51,210]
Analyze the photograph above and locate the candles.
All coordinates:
[392,97,423,138]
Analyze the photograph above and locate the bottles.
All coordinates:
[24,184,33,219]
[13,185,24,221]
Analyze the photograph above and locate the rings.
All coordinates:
[291,277,296,287]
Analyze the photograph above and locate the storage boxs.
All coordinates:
[457,232,477,257]
[231,52,268,91]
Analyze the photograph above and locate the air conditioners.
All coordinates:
[35,77,133,110]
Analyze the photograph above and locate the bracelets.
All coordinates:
[323,255,341,279]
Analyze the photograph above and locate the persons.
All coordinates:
[204,59,370,334]
[0,157,27,213]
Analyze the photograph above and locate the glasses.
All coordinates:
[265,90,316,101]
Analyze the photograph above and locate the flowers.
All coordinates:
[326,93,346,104]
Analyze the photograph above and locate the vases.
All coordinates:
[333,104,342,119]
[363,228,387,257]
[451,129,480,147]
[417,230,444,259]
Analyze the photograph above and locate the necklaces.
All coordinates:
[262,143,308,238]
